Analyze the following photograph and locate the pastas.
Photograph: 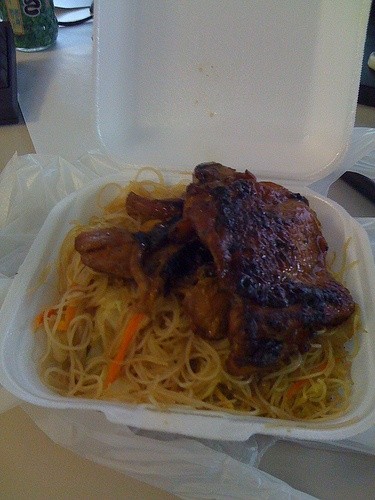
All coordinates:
[32,165,364,423]
